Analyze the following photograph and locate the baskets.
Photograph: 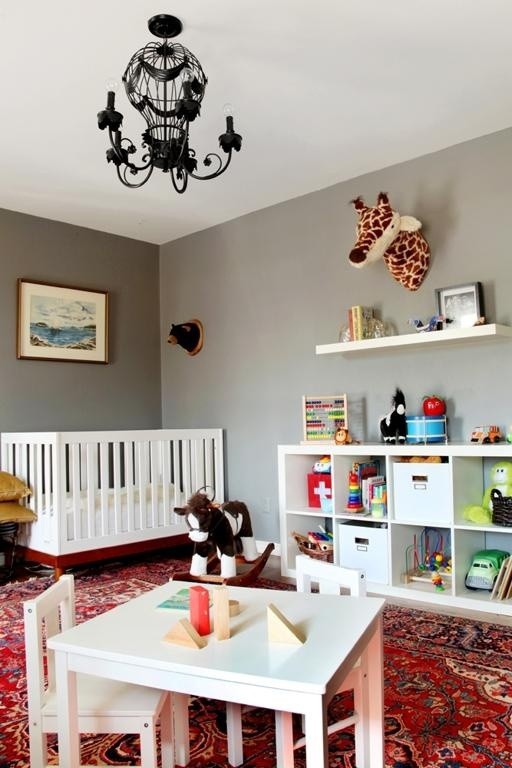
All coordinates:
[292,533,332,562]
[490,489,512,527]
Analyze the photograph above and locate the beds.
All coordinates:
[0,428,227,580]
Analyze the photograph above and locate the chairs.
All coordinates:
[226,554,368,768]
[23,574,174,768]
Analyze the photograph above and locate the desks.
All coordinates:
[46,578,387,768]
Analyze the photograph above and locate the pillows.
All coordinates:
[0,471,33,501]
[0,501,37,524]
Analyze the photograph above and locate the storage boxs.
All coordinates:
[337,520,390,586]
[392,462,453,525]
[305,472,333,510]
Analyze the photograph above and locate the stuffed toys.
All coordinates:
[346,191,430,291]
[446,461,512,525]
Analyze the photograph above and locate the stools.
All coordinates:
[0,522,20,582]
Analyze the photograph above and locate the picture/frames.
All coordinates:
[16,277,109,365]
[434,281,487,330]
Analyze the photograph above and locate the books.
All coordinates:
[347,457,387,518]
[348,304,373,341]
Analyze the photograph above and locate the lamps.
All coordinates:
[96,13,243,194]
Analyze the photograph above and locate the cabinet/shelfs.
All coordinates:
[277,442,512,627]
